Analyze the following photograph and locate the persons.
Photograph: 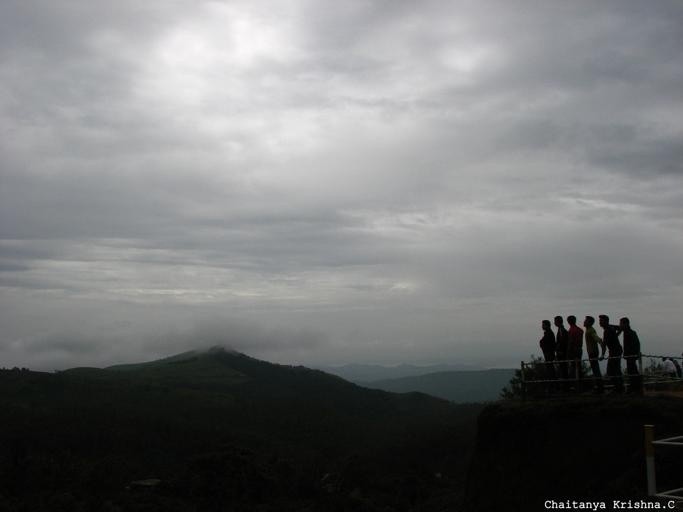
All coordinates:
[582,315,605,378]
[597,314,623,376]
[537,318,554,367]
[660,356,676,377]
[553,315,569,377]
[668,358,681,379]
[565,316,583,391]
[618,317,640,374]
